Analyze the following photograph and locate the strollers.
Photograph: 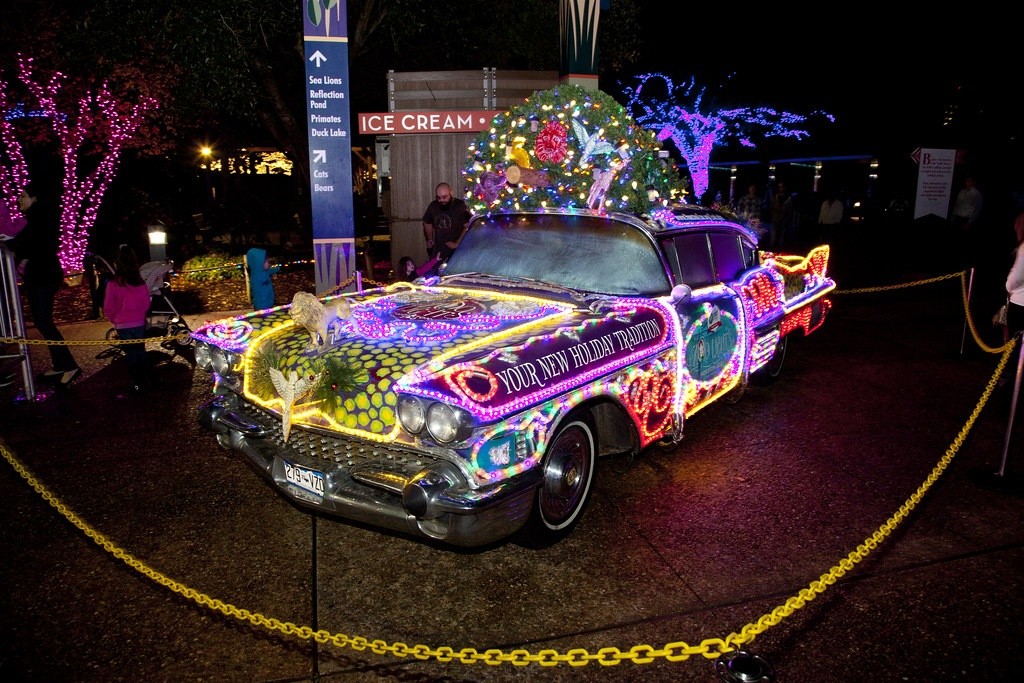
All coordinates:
[83,253,194,348]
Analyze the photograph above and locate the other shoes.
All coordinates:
[55,367,82,390]
[37,370,64,381]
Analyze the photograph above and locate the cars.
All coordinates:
[193,209,836,549]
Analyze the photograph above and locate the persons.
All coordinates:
[952,174,981,261]
[423,182,472,270]
[102,244,169,399]
[247,248,280,311]
[0,185,82,394]
[397,252,442,283]
[711,181,843,262]
[992,213,1024,423]
